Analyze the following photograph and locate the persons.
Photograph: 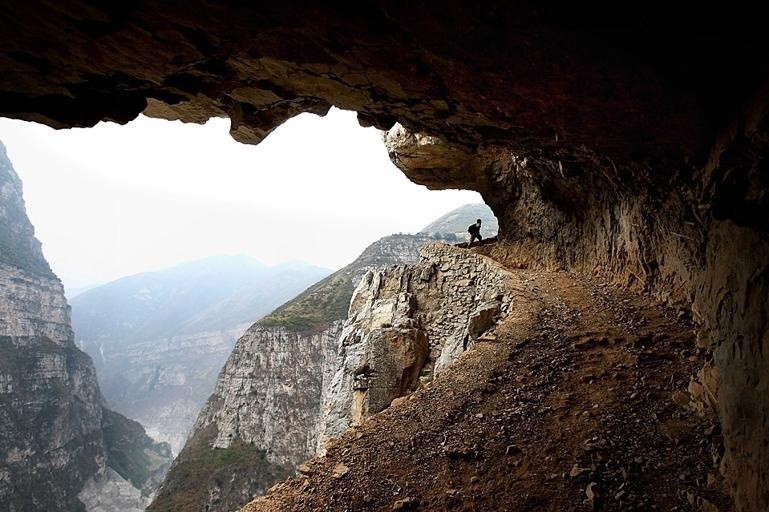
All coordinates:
[467,219,484,249]
